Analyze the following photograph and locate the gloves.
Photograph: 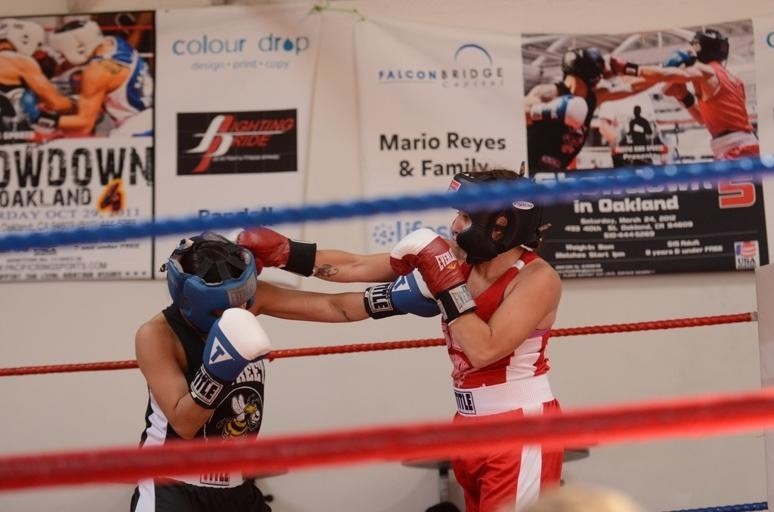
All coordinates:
[363,267,442,319]
[21,91,60,129]
[187,307,274,411]
[223,226,317,279]
[531,95,589,131]
[602,54,638,81]
[658,48,696,69]
[660,81,695,111]
[389,228,478,322]
[70,71,82,95]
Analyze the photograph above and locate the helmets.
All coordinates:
[0,17,45,55]
[685,29,730,67]
[445,168,553,264]
[161,228,258,335]
[47,20,106,66]
[563,45,613,89]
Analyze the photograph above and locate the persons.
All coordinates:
[0,18,77,143]
[525,47,698,174]
[611,30,760,163]
[130,229,441,512]
[236,169,565,512]
[20,20,154,137]
